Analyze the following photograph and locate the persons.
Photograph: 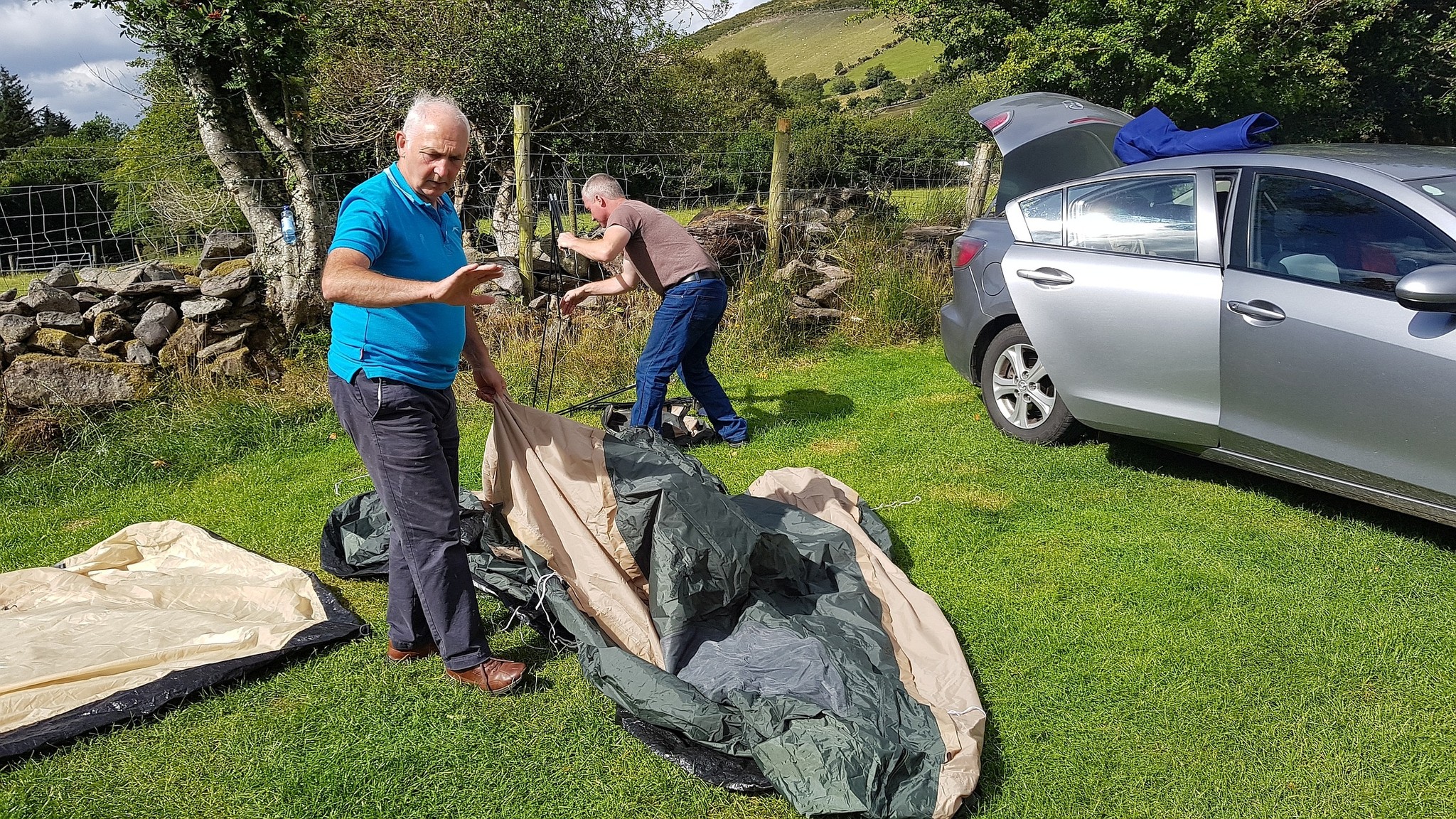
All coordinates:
[557,174,749,447]
[321,99,528,697]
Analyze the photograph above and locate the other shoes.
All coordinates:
[730,440,748,447]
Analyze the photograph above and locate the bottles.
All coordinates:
[281,205,296,243]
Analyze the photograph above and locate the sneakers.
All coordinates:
[385,639,436,662]
[446,656,526,695]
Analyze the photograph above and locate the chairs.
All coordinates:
[1267,207,1347,284]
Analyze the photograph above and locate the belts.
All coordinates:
[679,271,721,284]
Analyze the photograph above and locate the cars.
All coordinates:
[940,94,1456,532]
